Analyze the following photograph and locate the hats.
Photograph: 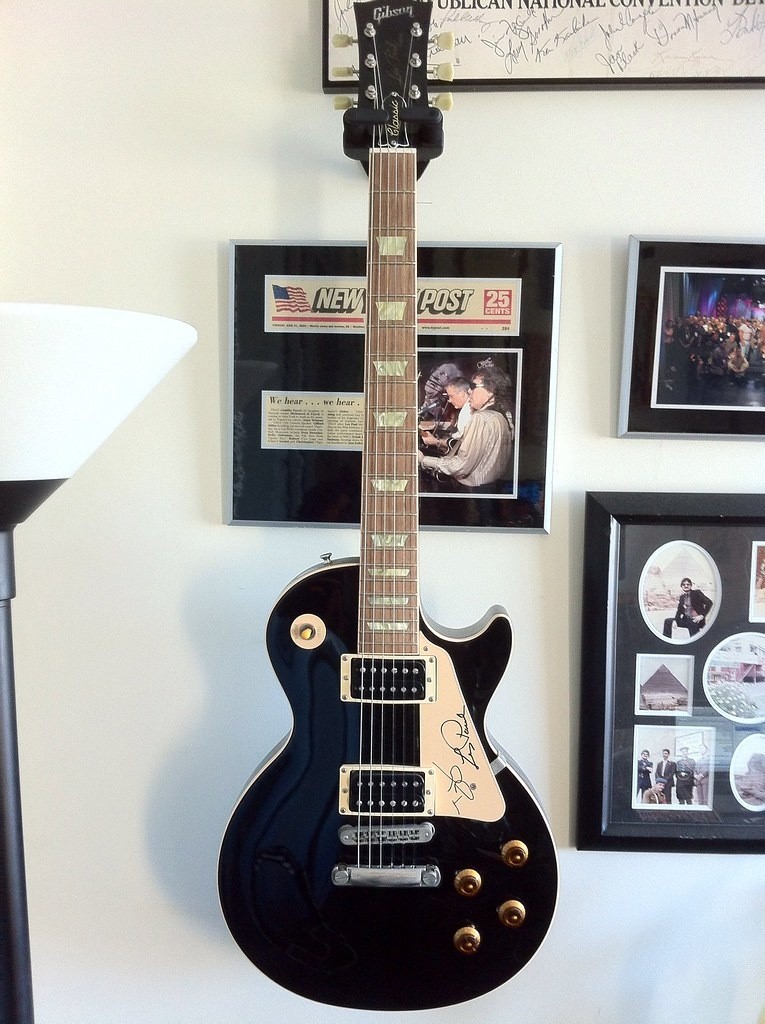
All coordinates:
[655,778,668,784]
[680,747,689,750]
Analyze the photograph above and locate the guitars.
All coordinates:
[216,1,559,1011]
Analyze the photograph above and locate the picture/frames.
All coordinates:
[217,236,566,536]
[616,234,765,441]
[322,0,765,92]
[574,492,765,855]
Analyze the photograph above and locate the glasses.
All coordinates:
[469,382,484,390]
[682,584,690,586]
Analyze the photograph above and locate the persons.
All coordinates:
[663,577,715,639]
[633,744,710,804]
[660,310,765,390]
[416,365,517,495]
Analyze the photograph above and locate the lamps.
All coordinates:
[0,300,197,1024]
